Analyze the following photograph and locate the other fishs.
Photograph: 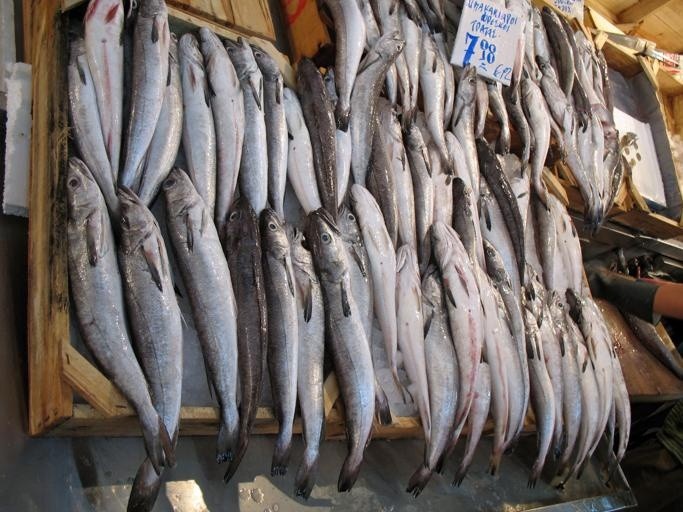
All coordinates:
[67,0,634,512]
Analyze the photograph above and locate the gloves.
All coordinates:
[585,257,661,327]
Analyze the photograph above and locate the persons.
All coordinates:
[589,267,683,323]
